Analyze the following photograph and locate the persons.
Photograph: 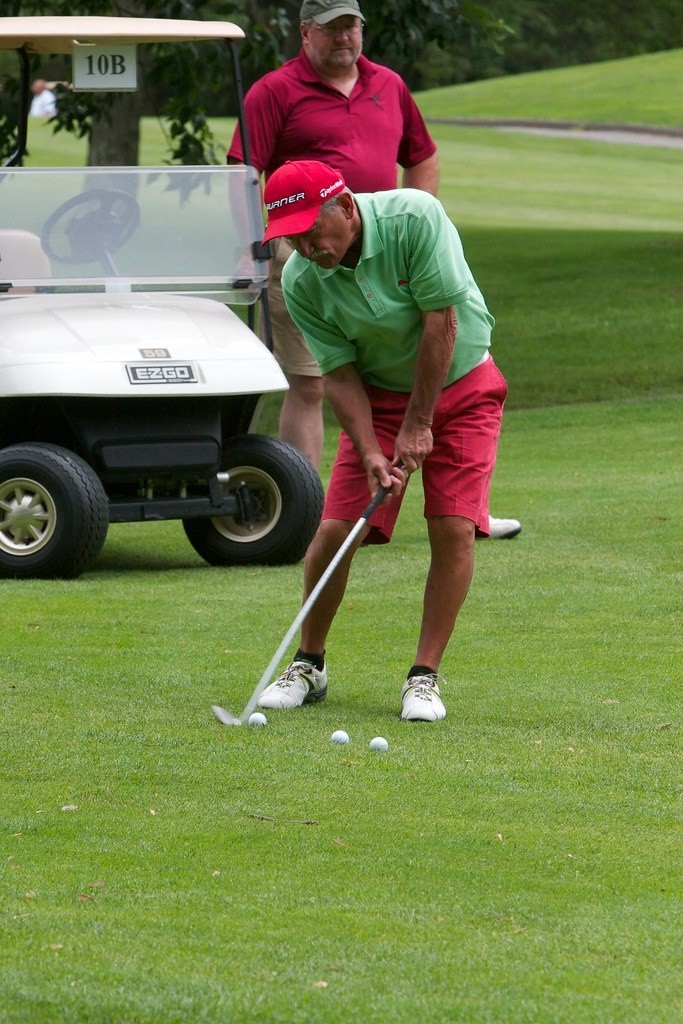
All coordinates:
[29,79,56,117]
[257,160,508,722]
[226,0,521,541]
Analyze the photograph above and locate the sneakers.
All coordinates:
[400,672,448,722]
[256,661,328,709]
[487,513,522,540]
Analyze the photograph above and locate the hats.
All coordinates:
[300,0,367,24]
[260,159,344,248]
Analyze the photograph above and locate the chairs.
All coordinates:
[0,228,52,295]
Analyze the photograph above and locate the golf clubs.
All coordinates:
[210,459,405,726]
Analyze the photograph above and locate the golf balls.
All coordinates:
[248,712,268,727]
[331,729,350,745]
[369,736,389,752]
